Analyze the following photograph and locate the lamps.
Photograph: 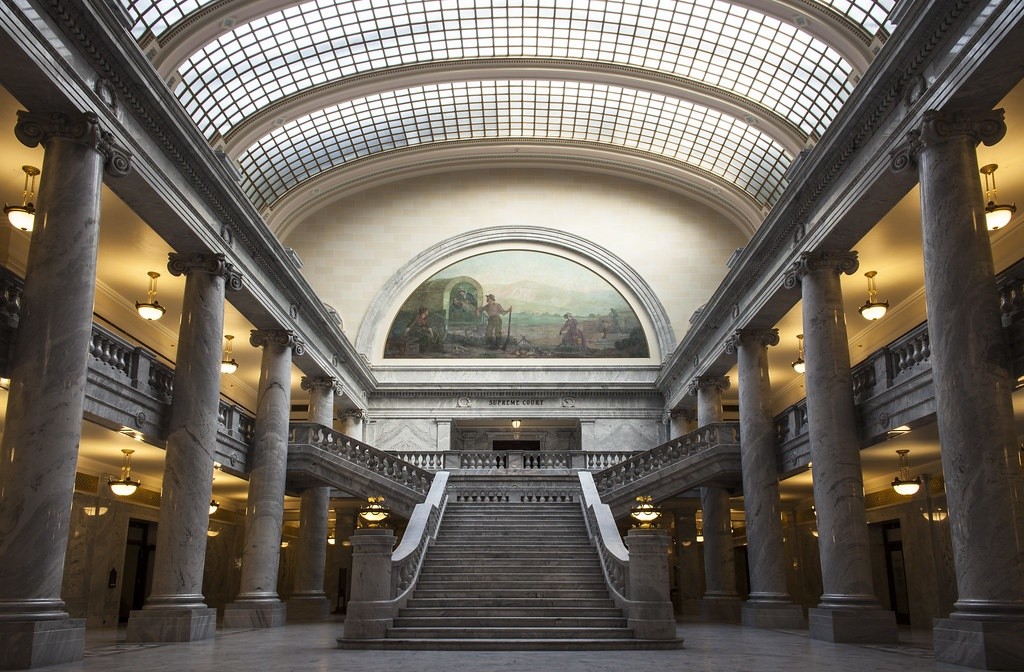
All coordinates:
[921,510,945,522]
[328,528,335,546]
[891,450,921,496]
[106,448,141,496]
[209,477,220,515]
[207,530,220,537]
[979,164,1016,230]
[791,334,805,374]
[511,420,521,429]
[3,164,41,232]
[280,541,288,547]
[221,335,239,373]
[630,495,662,529]
[358,496,391,529]
[695,519,704,542]
[859,272,889,321]
[135,272,166,321]
[83,504,107,516]
